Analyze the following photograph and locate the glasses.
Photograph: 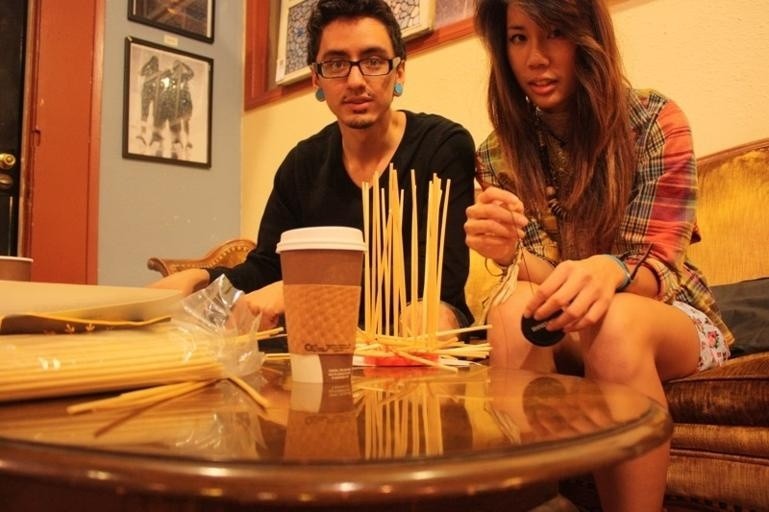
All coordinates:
[312,57,402,79]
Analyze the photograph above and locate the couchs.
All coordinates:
[143,137,769,510]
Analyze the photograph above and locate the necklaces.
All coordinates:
[535,116,583,224]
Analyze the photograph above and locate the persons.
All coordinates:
[465,0,732,511]
[146,0,476,344]
[149,65,186,158]
[488,367,645,450]
[248,374,476,463]
[170,57,195,150]
[133,52,165,147]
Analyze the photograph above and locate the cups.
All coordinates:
[275,226,368,384]
[283,385,362,460]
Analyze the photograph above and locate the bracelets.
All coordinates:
[493,238,523,270]
[611,255,632,290]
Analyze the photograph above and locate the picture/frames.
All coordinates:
[126,0,217,45]
[124,36,215,169]
[272,0,433,89]
